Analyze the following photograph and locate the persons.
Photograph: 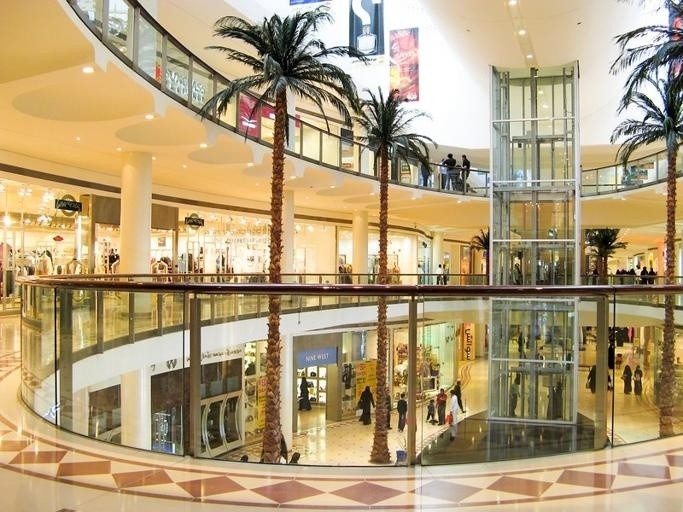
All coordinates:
[587,364,595,394]
[606,372,611,391]
[385,387,392,430]
[396,393,407,433]
[621,365,632,395]
[512,261,558,285]
[509,377,520,418]
[442,264,447,285]
[437,158,446,189]
[452,378,465,414]
[632,364,642,396]
[424,399,435,424]
[591,262,657,285]
[420,156,430,187]
[297,377,312,412]
[455,154,470,193]
[238,454,249,462]
[435,388,447,427]
[554,380,562,418]
[517,331,526,360]
[417,264,424,285]
[441,152,455,191]
[448,389,458,442]
[546,386,555,420]
[435,263,444,285]
[287,452,300,464]
[357,385,375,425]
